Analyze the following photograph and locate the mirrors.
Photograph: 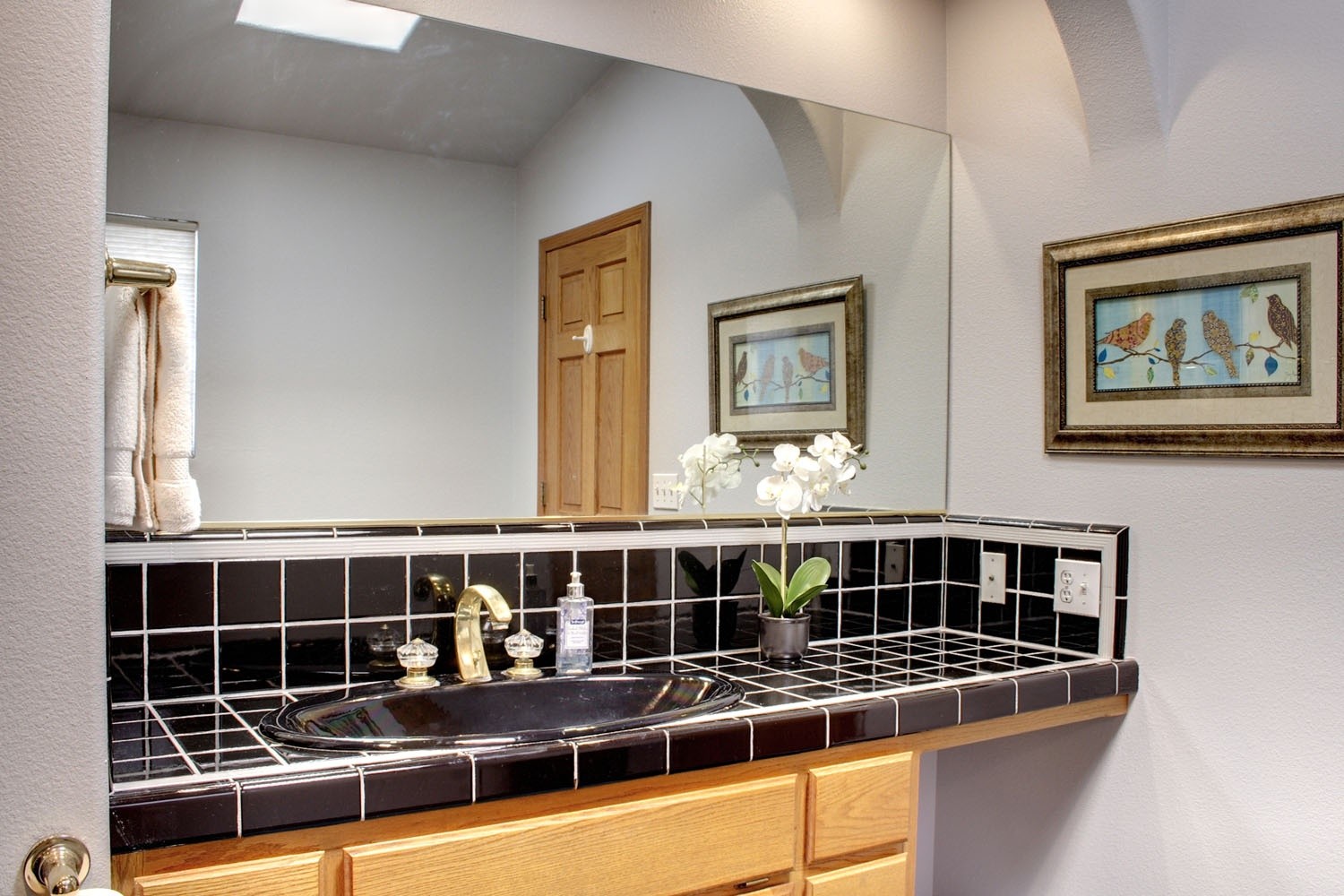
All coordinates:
[103,0,956,530]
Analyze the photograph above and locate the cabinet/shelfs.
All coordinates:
[110,739,925,896]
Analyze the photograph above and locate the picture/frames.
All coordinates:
[1040,193,1344,462]
[707,271,869,461]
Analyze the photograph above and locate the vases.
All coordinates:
[757,609,812,666]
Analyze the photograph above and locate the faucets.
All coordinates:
[453,583,512,680]
[411,572,458,673]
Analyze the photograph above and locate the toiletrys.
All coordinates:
[554,570,597,676]
[512,562,548,644]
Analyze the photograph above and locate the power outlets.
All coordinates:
[1053,558,1100,617]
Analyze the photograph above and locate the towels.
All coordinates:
[103,283,202,538]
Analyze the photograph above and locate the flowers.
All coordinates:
[668,432,761,516]
[752,428,870,616]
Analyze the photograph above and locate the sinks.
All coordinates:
[205,635,428,680]
[257,671,746,750]
